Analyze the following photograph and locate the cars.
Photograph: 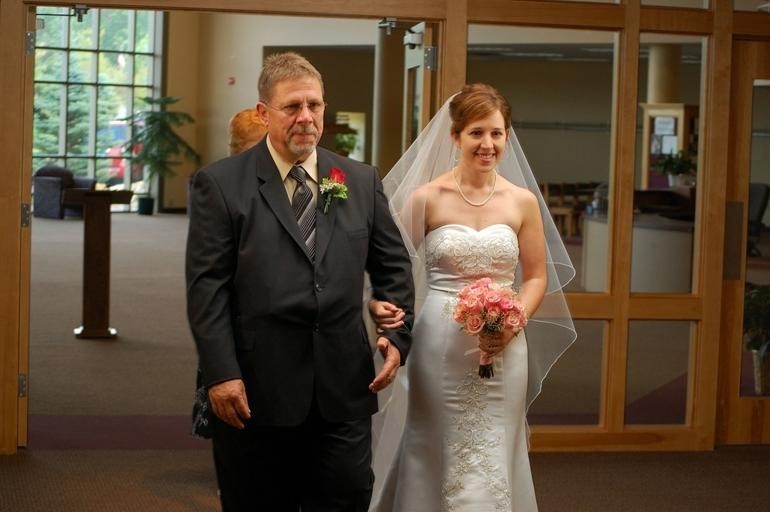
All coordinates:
[96,121,146,187]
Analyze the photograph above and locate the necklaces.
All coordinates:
[451,166,499,208]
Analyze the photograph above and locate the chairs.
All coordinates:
[543,182,595,244]
[33,164,97,220]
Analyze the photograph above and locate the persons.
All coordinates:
[187,106,270,440]
[361,83,552,511]
[184,51,416,511]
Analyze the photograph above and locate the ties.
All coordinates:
[289,166,317,262]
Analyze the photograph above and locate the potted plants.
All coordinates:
[649,147,692,188]
[744,285,770,396]
[115,97,202,214]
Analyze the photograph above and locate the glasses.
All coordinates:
[266,98,327,113]
